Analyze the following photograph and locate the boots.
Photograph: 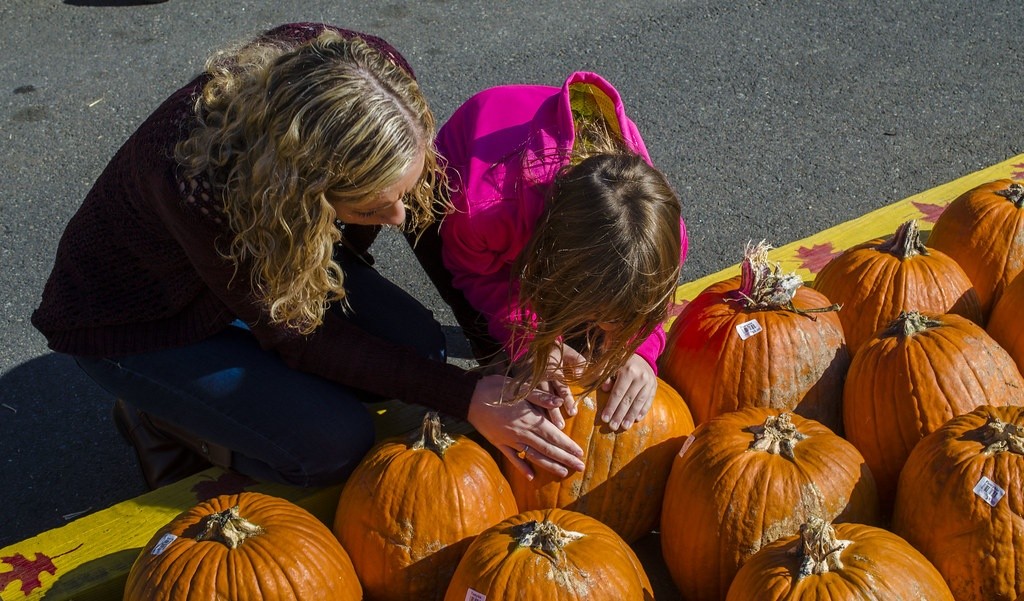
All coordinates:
[113,398,231,491]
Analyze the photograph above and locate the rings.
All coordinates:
[517,445,529,461]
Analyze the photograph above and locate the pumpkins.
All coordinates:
[122,178,1024,601]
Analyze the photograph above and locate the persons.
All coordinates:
[403,72,689,432]
[33,23,585,489]
